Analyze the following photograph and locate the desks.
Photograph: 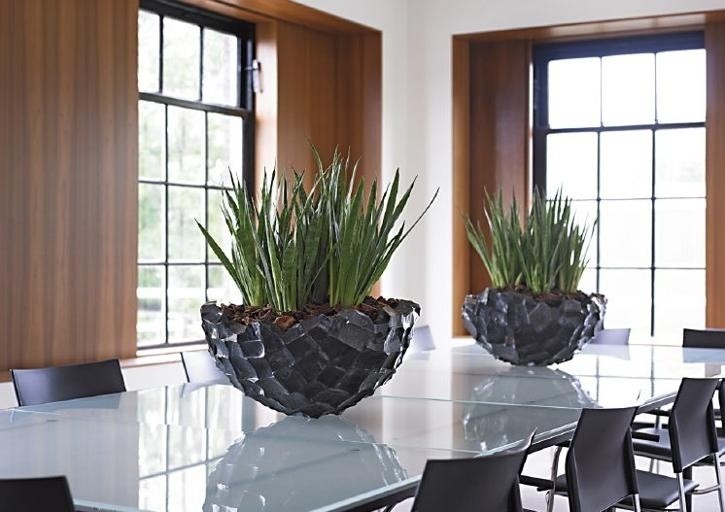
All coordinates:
[0,338,724,512]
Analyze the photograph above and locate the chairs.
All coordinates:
[412,428,538,512]
[0,474,76,511]
[682,328,725,348]
[548,377,723,510]
[630,379,725,472]
[180,349,226,382]
[589,325,629,346]
[6,358,127,409]
[519,391,646,512]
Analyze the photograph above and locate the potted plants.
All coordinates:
[459,182,611,368]
[196,136,441,423]
